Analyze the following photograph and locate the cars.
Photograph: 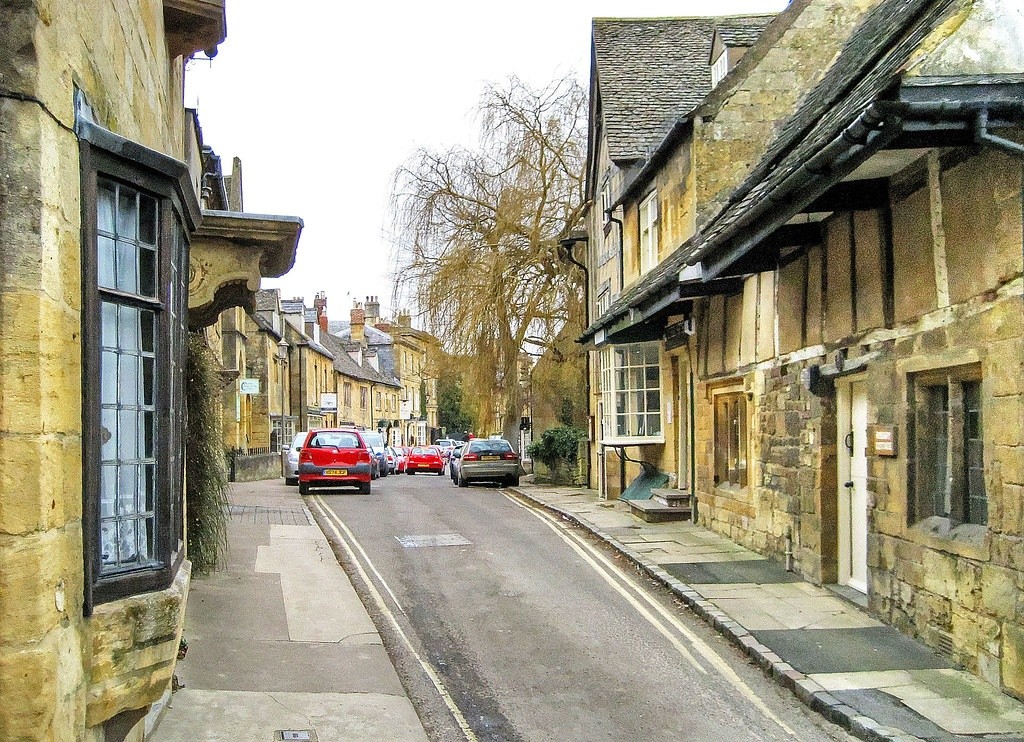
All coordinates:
[295,429,372,495]
[433,438,465,485]
[282,432,313,486]
[406,446,446,475]
[360,429,410,480]
[455,438,520,486]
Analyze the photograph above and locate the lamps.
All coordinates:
[274,337,289,366]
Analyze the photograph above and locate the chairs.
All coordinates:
[338,438,353,446]
[317,438,323,446]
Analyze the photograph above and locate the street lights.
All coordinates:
[276,336,288,478]
